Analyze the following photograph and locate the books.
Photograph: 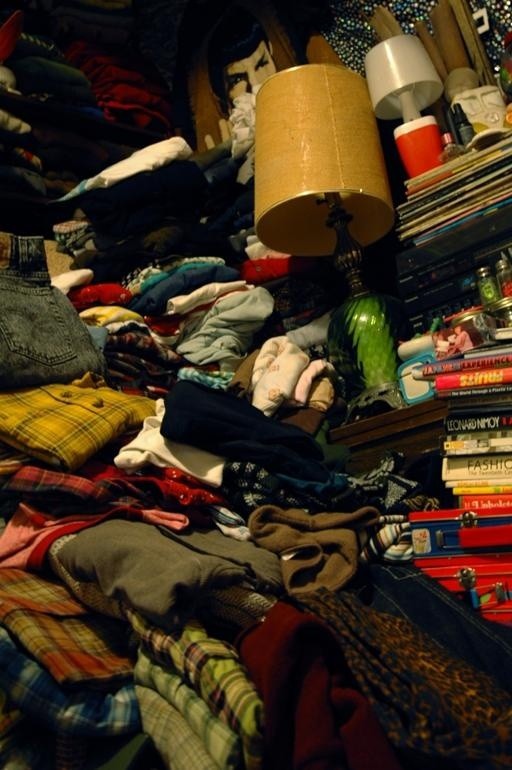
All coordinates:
[412,327,511,508]
[395,135,511,247]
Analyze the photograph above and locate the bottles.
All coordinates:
[441,102,474,162]
[476,259,512,334]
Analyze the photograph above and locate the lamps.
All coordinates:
[253,64,410,428]
[365,33,446,178]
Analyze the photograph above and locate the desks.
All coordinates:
[326,397,447,465]
